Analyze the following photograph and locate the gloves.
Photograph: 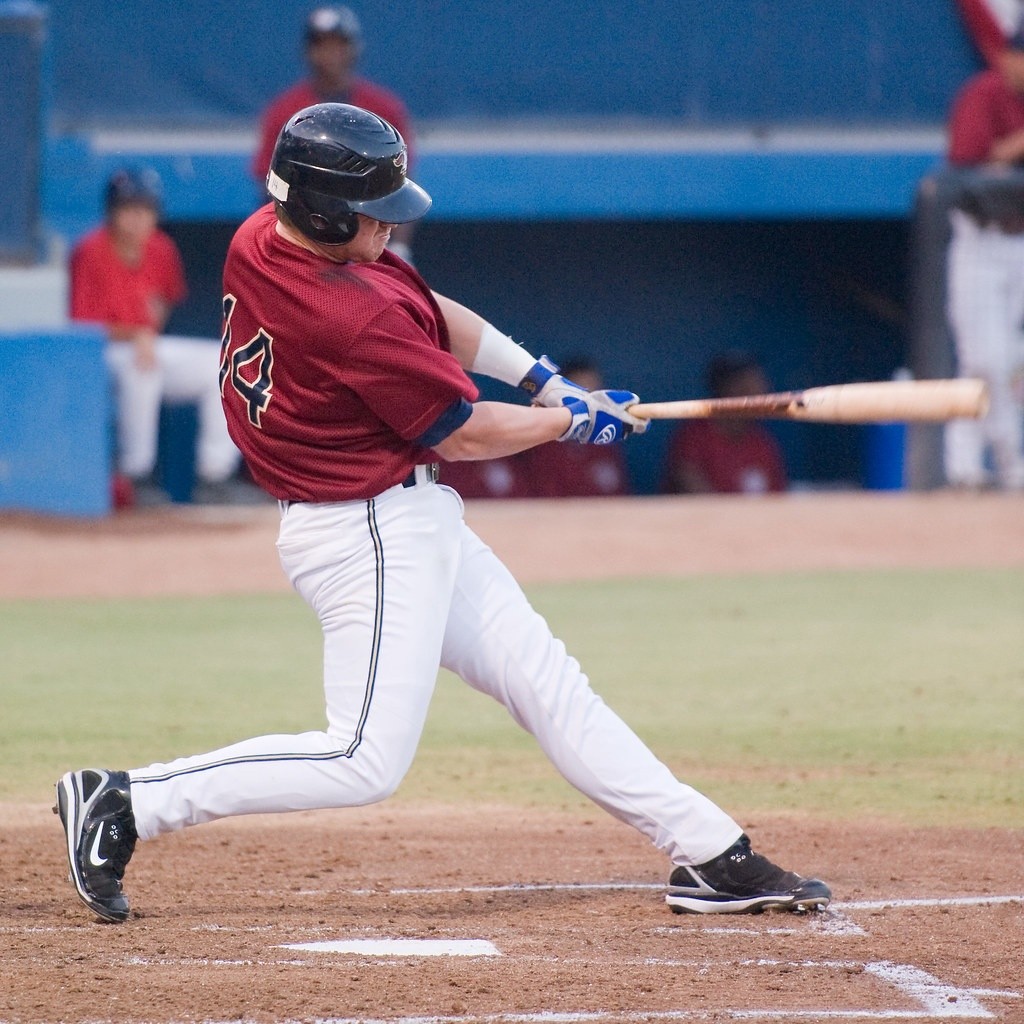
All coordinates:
[557,387,651,445]
[517,355,590,406]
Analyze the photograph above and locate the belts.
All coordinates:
[401,460,441,490]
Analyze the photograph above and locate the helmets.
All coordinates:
[302,6,361,39]
[265,101,433,245]
[109,166,167,211]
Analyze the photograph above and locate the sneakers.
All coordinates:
[54,768,137,923]
[664,831,832,914]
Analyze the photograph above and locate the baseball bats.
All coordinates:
[621,374,992,424]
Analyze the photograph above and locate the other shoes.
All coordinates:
[132,479,172,509]
[191,478,245,504]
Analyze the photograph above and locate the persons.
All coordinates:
[68,171,243,507]
[57,105,831,923]
[941,34,1024,494]
[670,360,790,496]
[427,357,627,501]
[251,7,414,267]
